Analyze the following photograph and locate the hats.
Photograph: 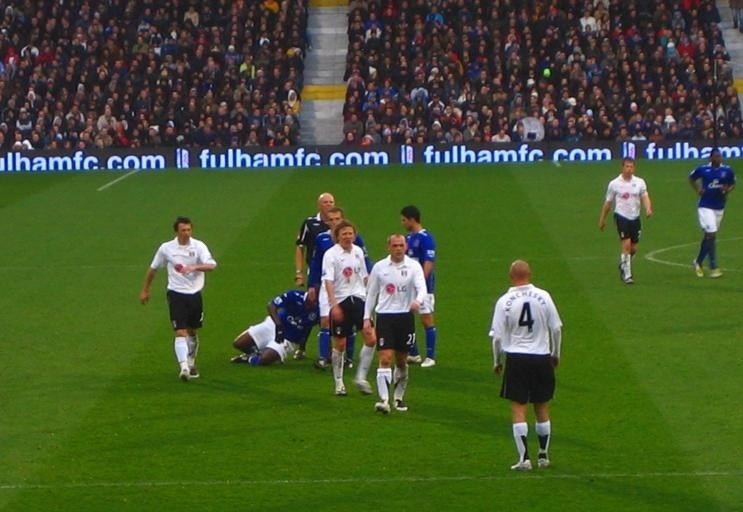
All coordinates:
[432,121,441,129]
[569,98,576,106]
[287,49,295,57]
[431,67,439,75]
[664,116,675,123]
[369,66,376,75]
[527,79,535,87]
[150,125,159,132]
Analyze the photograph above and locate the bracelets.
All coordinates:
[295,270,303,273]
[294,275,303,280]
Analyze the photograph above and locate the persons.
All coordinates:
[686,148,736,279]
[305,207,373,368]
[320,220,376,398]
[227,285,321,367]
[292,192,335,287]
[137,216,216,381]
[597,156,652,284]
[400,205,436,367]
[0,0,307,155]
[361,232,428,415]
[487,259,564,471]
[344,1,743,144]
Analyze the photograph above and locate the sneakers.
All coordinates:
[511,459,532,471]
[352,377,373,395]
[180,368,189,381]
[374,401,391,415]
[538,459,550,469]
[189,367,200,378]
[620,271,633,283]
[335,381,347,395]
[693,259,704,277]
[314,358,328,371]
[231,353,249,363]
[393,399,408,411]
[406,355,421,363]
[710,268,722,278]
[421,358,436,368]
[293,349,305,360]
